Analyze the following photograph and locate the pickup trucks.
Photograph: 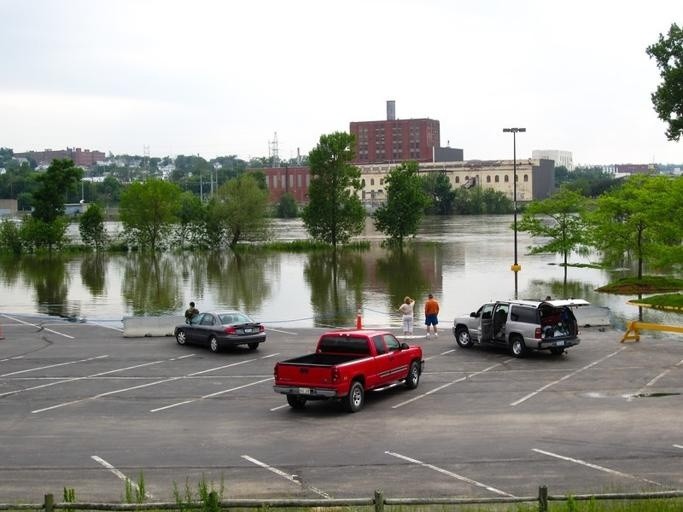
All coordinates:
[271,330,425,411]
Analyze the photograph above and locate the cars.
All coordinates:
[173,310,268,351]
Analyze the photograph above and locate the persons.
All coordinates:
[399,296,416,335]
[424,294,439,339]
[545,296,551,300]
[185,302,199,323]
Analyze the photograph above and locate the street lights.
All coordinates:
[213,161,223,198]
[79,178,85,203]
[504,127,528,297]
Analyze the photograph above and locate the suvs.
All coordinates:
[450,296,592,360]
